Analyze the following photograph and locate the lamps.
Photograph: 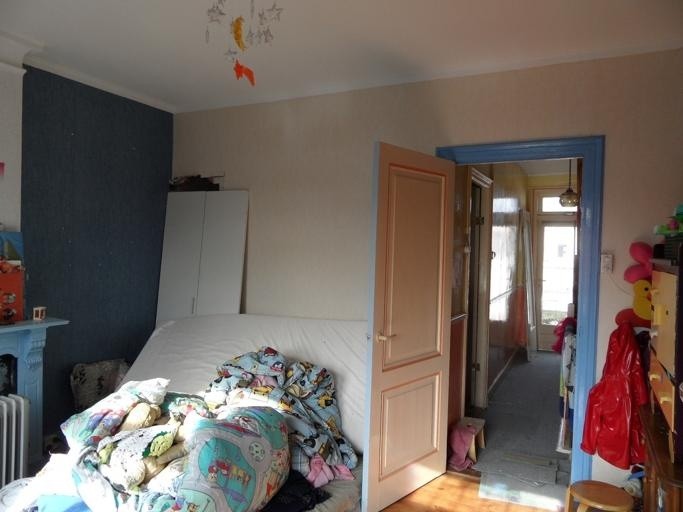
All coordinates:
[559,160,578,207]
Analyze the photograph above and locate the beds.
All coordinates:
[1,314,369,512]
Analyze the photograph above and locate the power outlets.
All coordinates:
[601,255,613,274]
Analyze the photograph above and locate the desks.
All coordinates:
[1,317,70,464]
[640,400,683,512]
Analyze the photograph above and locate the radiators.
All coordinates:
[0,394,30,489]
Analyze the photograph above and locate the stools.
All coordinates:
[458,417,487,462]
[565,481,635,512]
